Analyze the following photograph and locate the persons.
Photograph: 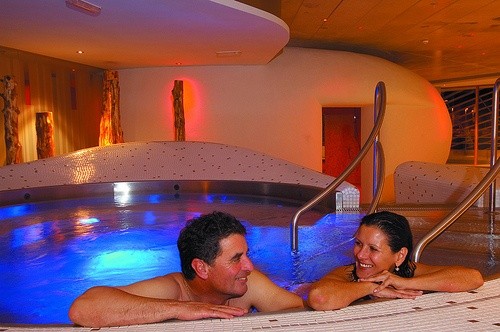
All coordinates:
[307,212,484,312]
[69,212,308,327]
